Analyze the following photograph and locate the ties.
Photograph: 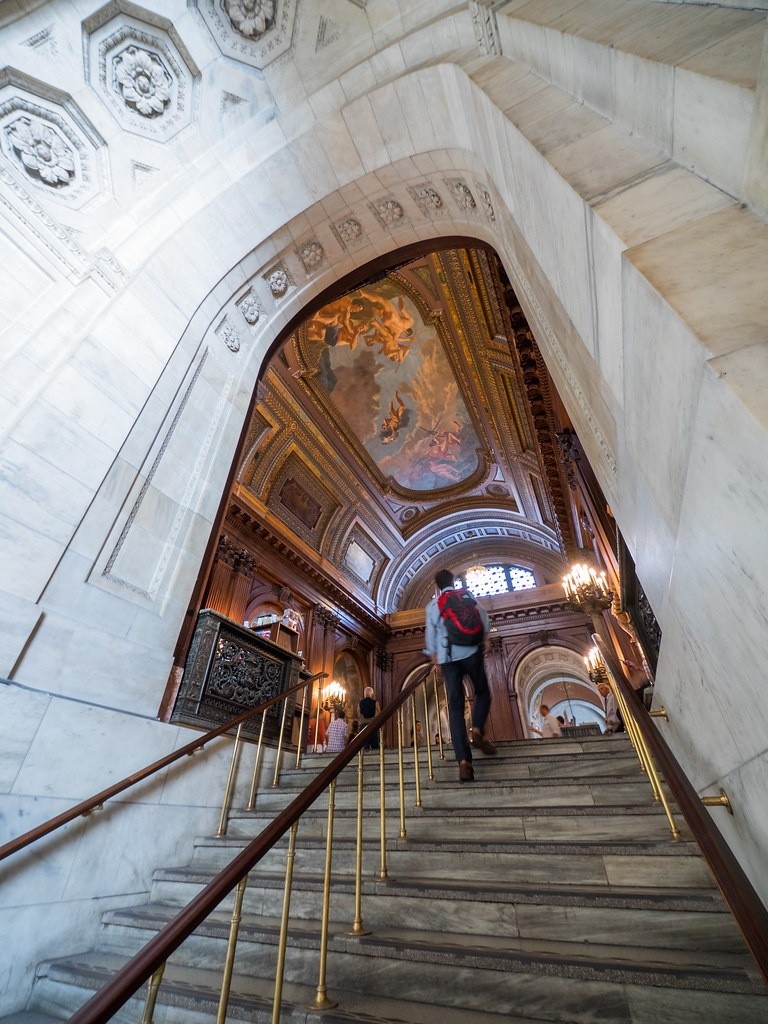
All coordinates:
[605,697,607,715]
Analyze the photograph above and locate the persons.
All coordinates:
[425,569,498,783]
[325,711,359,753]
[434,733,446,746]
[359,687,381,752]
[598,683,622,735]
[408,720,425,747]
[529,705,566,738]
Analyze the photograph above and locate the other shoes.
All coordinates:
[467,730,497,756]
[459,764,475,780]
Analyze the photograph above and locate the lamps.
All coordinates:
[584,647,608,684]
[321,681,346,713]
[562,563,614,614]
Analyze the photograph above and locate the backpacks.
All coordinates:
[436,588,484,659]
